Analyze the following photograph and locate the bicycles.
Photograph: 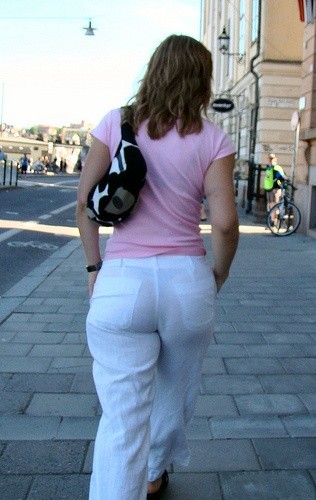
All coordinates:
[266,179,301,238]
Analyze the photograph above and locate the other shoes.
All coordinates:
[200,215,207,221]
[271,222,276,226]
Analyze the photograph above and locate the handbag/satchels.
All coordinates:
[86,106,147,227]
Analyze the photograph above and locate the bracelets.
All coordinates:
[86,260,103,273]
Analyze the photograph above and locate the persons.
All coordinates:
[75,34,241,500]
[264,154,286,227]
[0,147,83,176]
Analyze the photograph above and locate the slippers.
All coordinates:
[147,469,169,499]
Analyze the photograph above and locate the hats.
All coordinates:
[269,154,276,159]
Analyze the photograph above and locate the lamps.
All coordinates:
[217,26,246,57]
